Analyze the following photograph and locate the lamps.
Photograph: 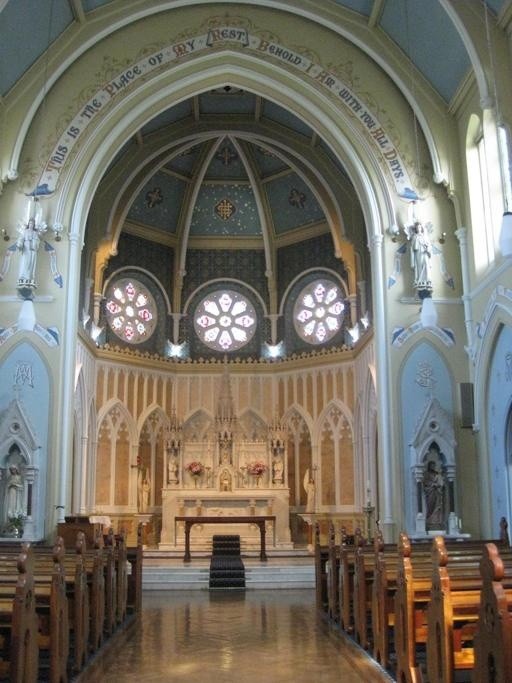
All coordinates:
[17,297,38,332]
[498,210,512,259]
[420,296,438,329]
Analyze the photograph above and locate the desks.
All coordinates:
[174,514,277,560]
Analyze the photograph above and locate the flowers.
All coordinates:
[248,462,267,476]
[186,461,205,476]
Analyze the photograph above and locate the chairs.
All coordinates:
[312,515,512,683]
[0,519,144,683]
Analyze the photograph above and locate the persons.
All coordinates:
[305,478,315,514]
[14,218,40,288]
[408,222,434,293]
[142,479,150,505]
[2,464,23,522]
[423,460,446,532]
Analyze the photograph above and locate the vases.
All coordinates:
[255,477,261,488]
[191,475,199,488]
